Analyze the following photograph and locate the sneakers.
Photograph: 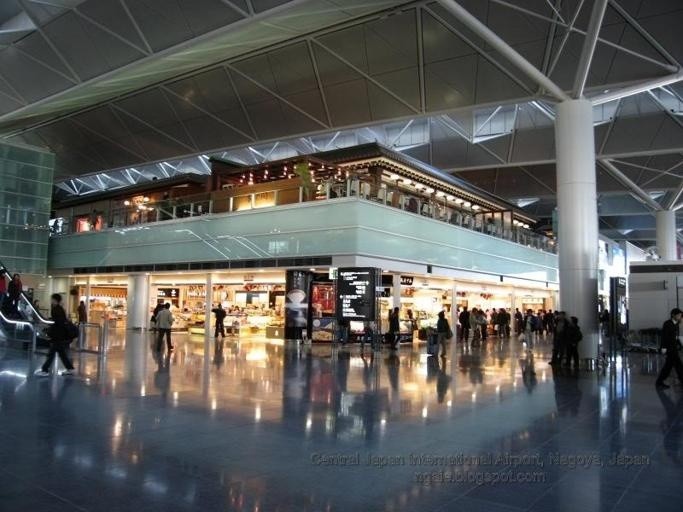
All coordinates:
[655,380,671,388]
[60,367,76,375]
[35,370,49,377]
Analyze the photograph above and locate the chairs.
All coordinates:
[337,179,554,253]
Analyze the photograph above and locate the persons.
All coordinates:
[88,207,98,231]
[34,294,73,376]
[160,191,170,207]
[154,352,176,397]
[403,192,557,254]
[383,305,683,391]
[337,351,377,395]
[33,300,40,310]
[360,321,376,349]
[6,273,22,314]
[151,301,241,353]
[76,300,87,322]
[430,356,453,404]
[173,194,185,218]
[336,318,350,346]
[210,338,229,372]
[0,269,6,308]
